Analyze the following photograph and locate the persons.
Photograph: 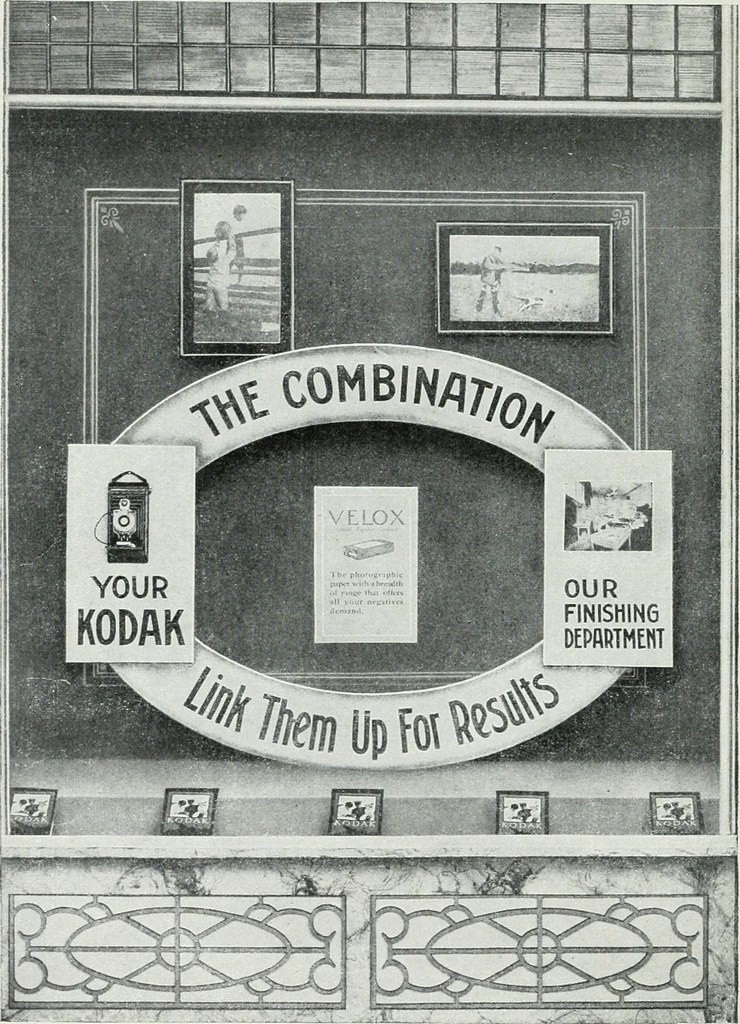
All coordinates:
[475,246,507,317]
[336,800,376,822]
[656,800,694,821]
[204,230,237,311]
[12,796,41,816]
[505,801,542,823]
[170,798,205,818]
[228,204,248,283]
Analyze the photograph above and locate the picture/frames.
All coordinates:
[9,787,59,836]
[178,176,296,359]
[160,786,219,834]
[435,220,616,337]
[326,788,386,835]
[648,791,707,836]
[495,788,551,836]
